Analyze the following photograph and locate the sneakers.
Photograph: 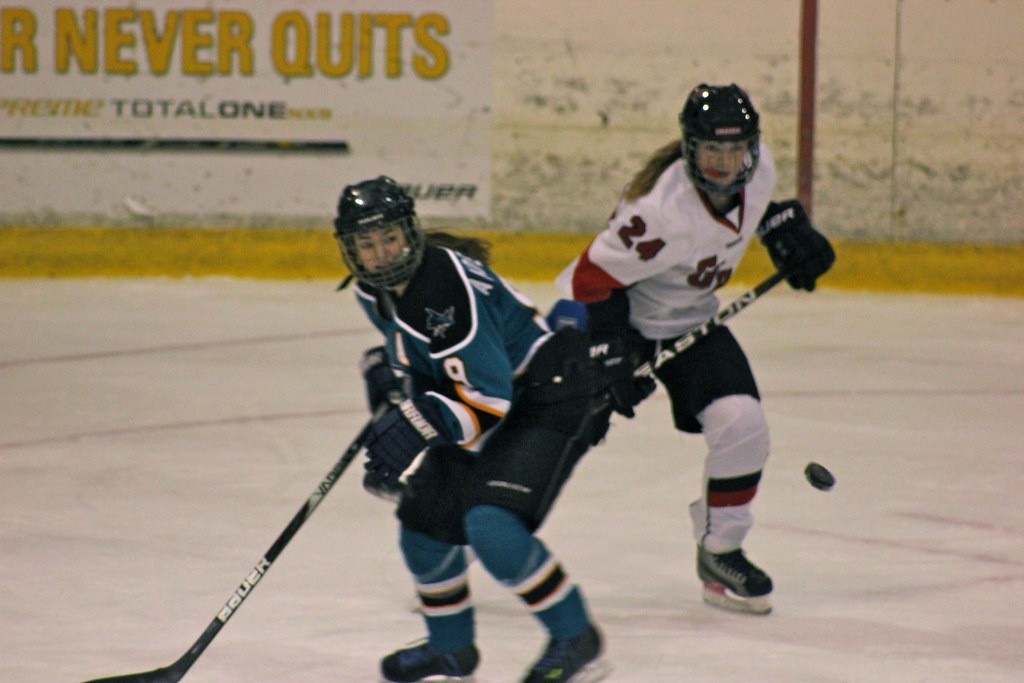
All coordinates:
[697,545,774,615]
[381,643,481,683]
[522,625,617,683]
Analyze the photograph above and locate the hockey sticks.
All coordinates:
[595,266,787,414]
[83,400,390,683]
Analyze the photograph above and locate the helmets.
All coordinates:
[678,83,761,198]
[333,176,425,290]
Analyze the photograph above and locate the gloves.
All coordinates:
[364,398,453,492]
[758,199,836,292]
[583,290,656,419]
[360,346,407,421]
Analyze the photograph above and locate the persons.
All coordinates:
[543,82,837,617]
[333,176,612,682]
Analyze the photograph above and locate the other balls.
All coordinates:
[804,461,834,491]
